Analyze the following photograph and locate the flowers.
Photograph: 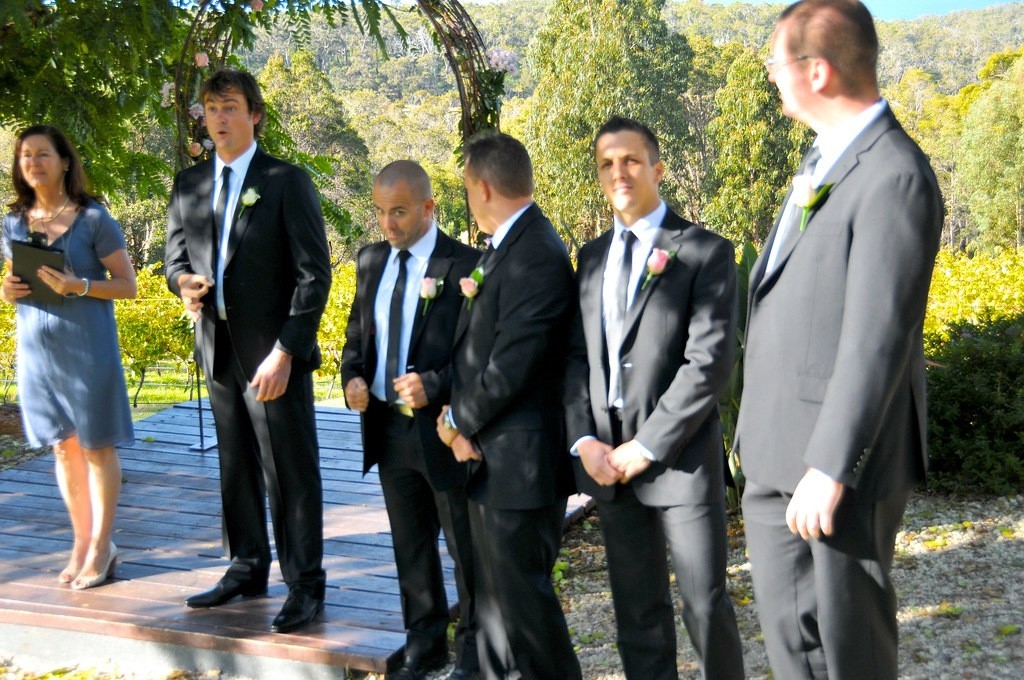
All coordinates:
[420,276,447,316]
[238,186,261,218]
[159,0,518,166]
[640,248,676,290]
[459,264,486,311]
[790,175,834,231]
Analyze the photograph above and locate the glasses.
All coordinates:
[765,55,813,72]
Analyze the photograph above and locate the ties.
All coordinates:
[766,142,822,273]
[385,248,412,406]
[617,228,637,326]
[475,242,494,275]
[215,164,232,254]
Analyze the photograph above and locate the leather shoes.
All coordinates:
[270,581,327,635]
[186,568,270,608]
[385,654,449,680]
[449,663,480,680]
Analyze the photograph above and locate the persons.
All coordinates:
[564,115,745,680]
[165,70,330,631]
[730,0,946,679]
[0,124,136,589]
[340,160,482,680]
[436,133,582,680]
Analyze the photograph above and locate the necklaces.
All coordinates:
[26,196,69,222]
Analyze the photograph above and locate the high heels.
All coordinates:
[60,537,119,590]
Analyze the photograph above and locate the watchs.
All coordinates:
[442,409,454,430]
[78,277,90,296]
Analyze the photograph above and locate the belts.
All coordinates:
[374,401,429,417]
